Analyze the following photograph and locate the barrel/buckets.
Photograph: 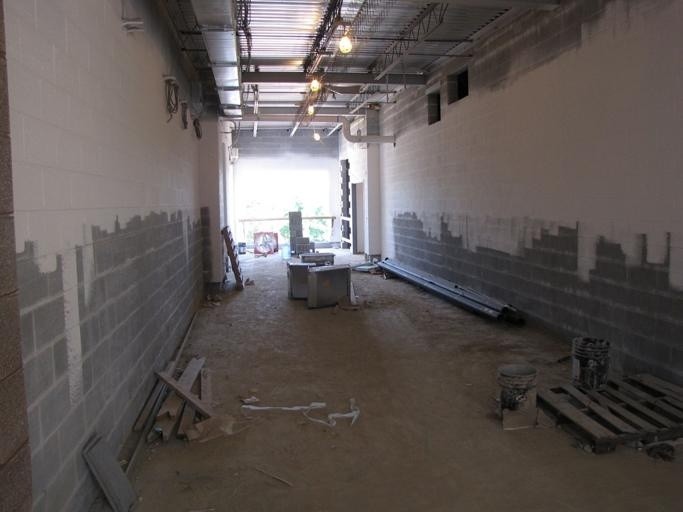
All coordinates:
[498,362,539,423]
[572,334,612,392]
[239,242,246,254]
